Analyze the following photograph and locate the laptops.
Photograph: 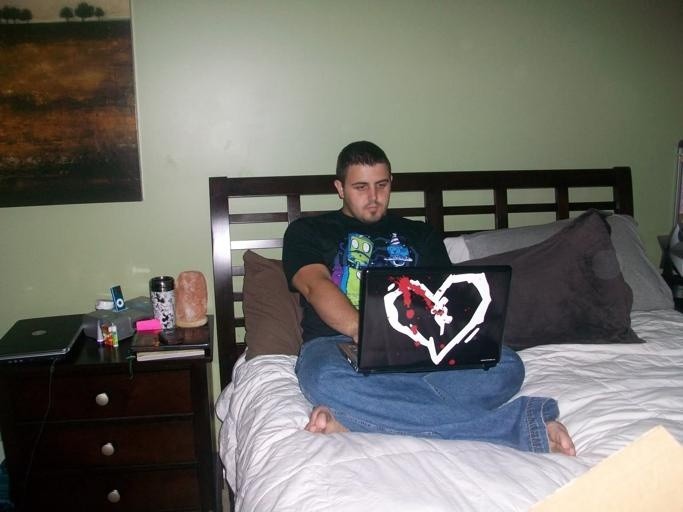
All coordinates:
[336,266,511,375]
[0,314,87,364]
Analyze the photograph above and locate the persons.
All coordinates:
[282,141,577,456]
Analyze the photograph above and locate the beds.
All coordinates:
[208,166,682,509]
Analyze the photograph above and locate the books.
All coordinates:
[136,349,205,361]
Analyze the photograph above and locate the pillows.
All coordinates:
[242,248,303,362]
[444,214,674,314]
[450,210,646,353]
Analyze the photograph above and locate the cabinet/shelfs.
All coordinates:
[0,314,223,511]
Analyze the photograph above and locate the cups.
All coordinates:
[148,275,178,334]
